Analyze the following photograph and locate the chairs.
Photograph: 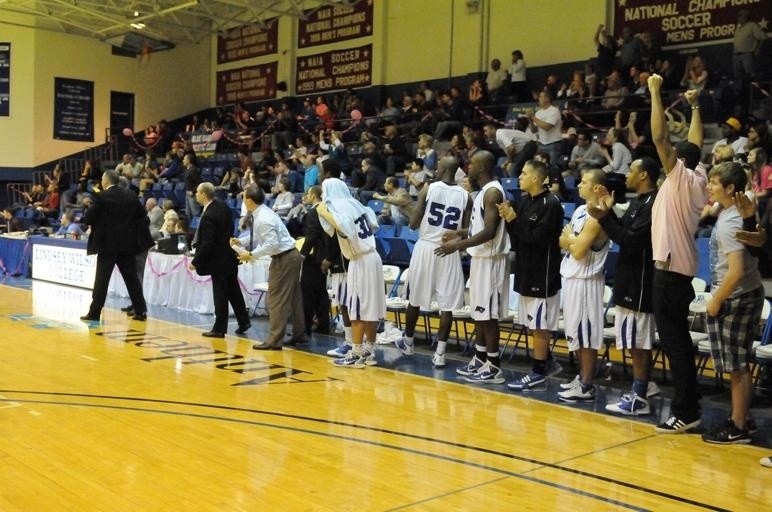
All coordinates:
[2,100,301,320]
[488,70,771,391]
[299,75,490,358]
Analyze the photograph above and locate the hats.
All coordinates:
[723,117,741,131]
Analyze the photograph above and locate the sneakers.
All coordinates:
[546,358,563,379]
[606,382,659,416]
[326,343,377,369]
[455,356,484,375]
[507,373,549,393]
[560,374,581,390]
[395,337,418,356]
[431,352,446,368]
[656,410,703,433]
[761,455,772,467]
[557,387,596,402]
[703,416,757,444]
[464,360,505,384]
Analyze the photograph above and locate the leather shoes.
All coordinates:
[253,342,282,350]
[202,331,225,338]
[122,306,146,320]
[80,315,99,321]
[236,324,251,333]
[284,338,307,346]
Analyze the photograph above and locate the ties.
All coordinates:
[249,215,254,252]
